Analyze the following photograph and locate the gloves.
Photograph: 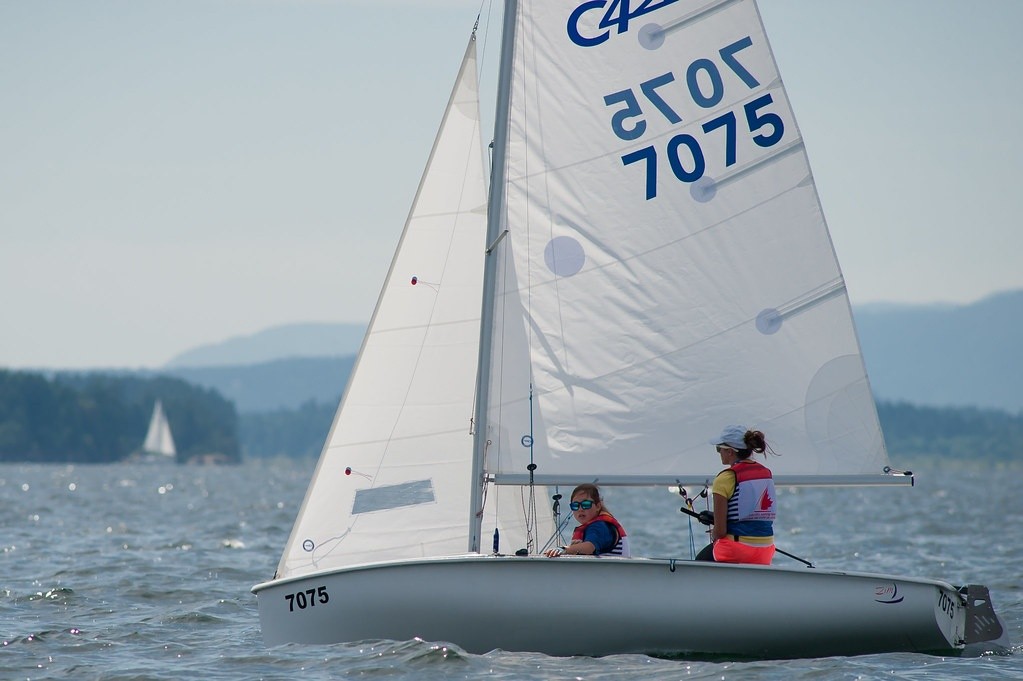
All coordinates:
[698,510,714,525]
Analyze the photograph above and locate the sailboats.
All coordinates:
[120,395,178,466]
[250,0,1011,661]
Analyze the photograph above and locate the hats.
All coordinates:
[709,425,750,449]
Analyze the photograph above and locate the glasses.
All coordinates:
[716,444,739,453]
[570,500,597,511]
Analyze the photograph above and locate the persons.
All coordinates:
[694,424,776,565]
[544,483,630,558]
